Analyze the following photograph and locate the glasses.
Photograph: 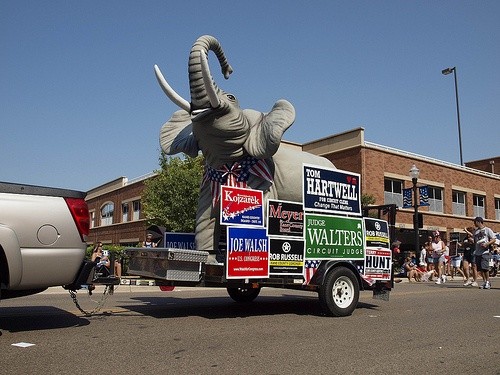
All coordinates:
[98,246,102,247]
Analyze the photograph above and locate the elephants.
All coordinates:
[154,35,338,265]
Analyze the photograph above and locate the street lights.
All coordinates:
[408,165,421,268]
[441,66,464,169]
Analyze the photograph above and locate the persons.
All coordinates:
[432,235,445,285]
[472,217,497,289]
[450,239,467,281]
[425,236,433,281]
[444,243,451,276]
[400,256,424,282]
[419,245,429,271]
[91,242,122,278]
[460,227,478,287]
[142,232,157,248]
[393,244,401,263]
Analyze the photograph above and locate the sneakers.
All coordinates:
[435,275,447,284]
[479,281,491,288]
[464,280,478,287]
[450,275,466,280]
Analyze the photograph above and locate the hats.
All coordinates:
[147,234,152,239]
[433,229,439,236]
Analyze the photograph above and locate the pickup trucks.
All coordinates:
[0,179,120,294]
